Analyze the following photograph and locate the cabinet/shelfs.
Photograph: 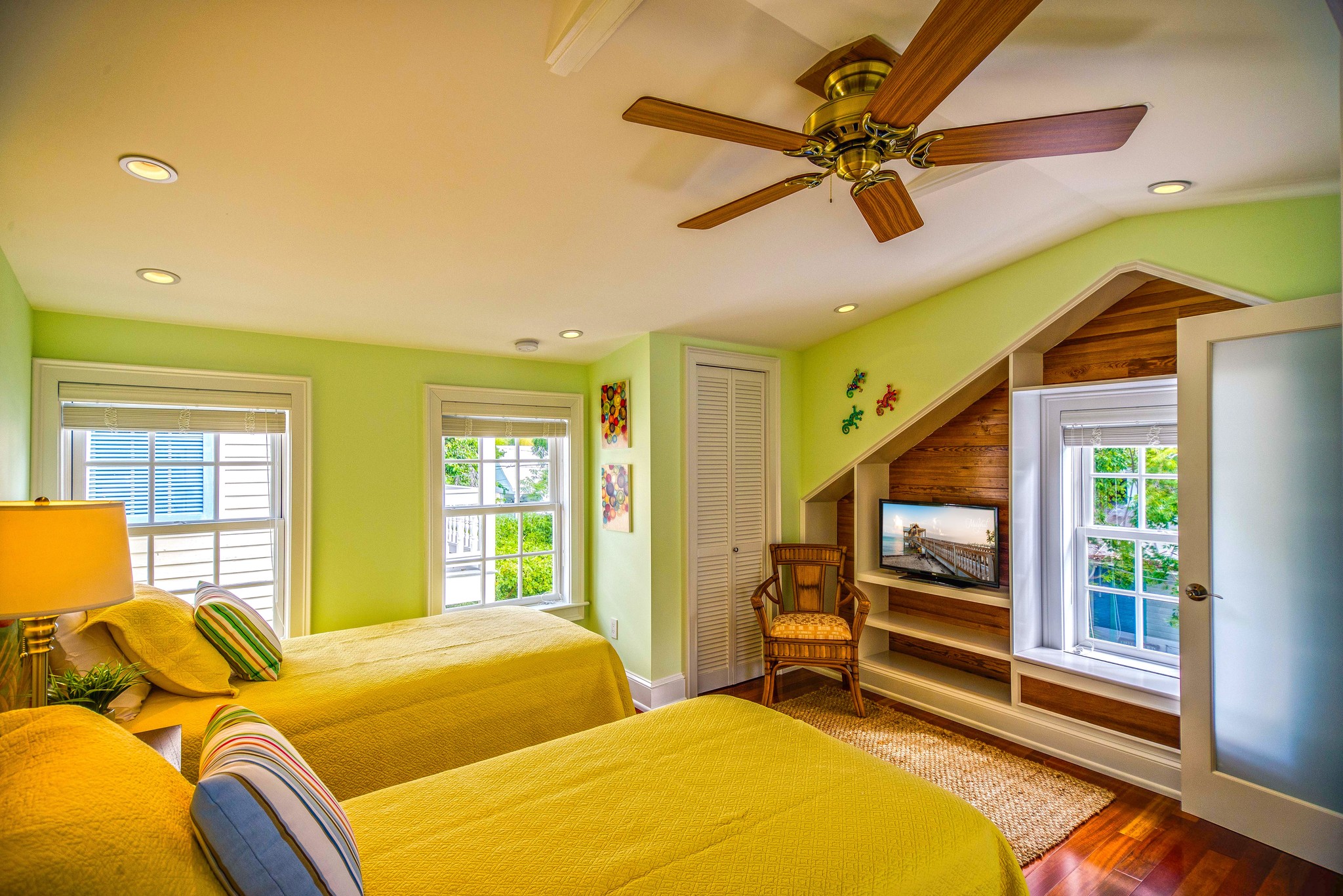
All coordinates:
[856,566,1014,711]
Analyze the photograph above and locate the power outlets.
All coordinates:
[610,617,618,639]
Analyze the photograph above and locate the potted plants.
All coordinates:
[7,653,164,723]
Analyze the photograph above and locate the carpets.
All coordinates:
[768,683,1116,871]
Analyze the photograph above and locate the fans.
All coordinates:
[621,3,1149,243]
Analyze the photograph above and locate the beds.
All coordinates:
[116,605,638,803]
[340,695,1029,896]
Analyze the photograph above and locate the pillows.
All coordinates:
[0,705,230,896]
[50,611,152,724]
[193,579,284,683]
[190,703,364,896]
[77,582,241,698]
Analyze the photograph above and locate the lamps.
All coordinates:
[514,339,539,353]
[0,496,135,712]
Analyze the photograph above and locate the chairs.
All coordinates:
[750,542,871,718]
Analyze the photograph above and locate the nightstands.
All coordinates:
[132,723,182,776]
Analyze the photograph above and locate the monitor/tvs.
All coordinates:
[878,498,999,588]
